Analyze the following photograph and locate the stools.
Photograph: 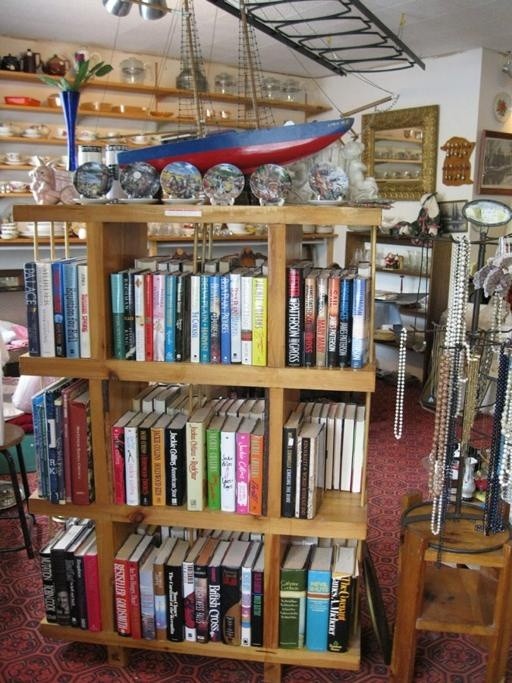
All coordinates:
[0,423,41,562]
[390,500,512,682]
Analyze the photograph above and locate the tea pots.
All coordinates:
[39,52,72,76]
[0,53,20,71]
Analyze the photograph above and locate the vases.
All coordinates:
[60,92,79,172]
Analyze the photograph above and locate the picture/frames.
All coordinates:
[475,129,512,194]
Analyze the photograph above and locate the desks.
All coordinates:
[1,317,29,443]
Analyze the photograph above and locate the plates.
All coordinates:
[161,198,201,204]
[119,198,159,204]
[149,111,173,116]
[308,200,346,205]
[202,163,245,199]
[53,135,67,139]
[250,164,292,201]
[74,162,113,198]
[21,135,42,138]
[120,162,160,197]
[77,137,95,141]
[131,141,150,145]
[160,161,202,198]
[0,159,32,165]
[20,222,73,238]
[309,163,349,199]
[73,198,115,205]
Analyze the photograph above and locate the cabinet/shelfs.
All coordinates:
[0,68,339,246]
[11,201,384,680]
[345,227,455,390]
[375,133,423,181]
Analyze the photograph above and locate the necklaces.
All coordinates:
[392,327,407,439]
[425,231,512,537]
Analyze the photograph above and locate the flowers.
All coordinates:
[35,52,114,91]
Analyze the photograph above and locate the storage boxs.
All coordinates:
[0,434,39,476]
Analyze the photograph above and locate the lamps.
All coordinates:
[104,1,171,19]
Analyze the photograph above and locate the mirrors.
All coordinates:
[359,105,439,200]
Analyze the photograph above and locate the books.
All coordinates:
[22,254,371,369]
[32,377,366,519]
[40,517,359,654]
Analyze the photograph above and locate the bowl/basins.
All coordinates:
[56,128,67,136]
[11,181,26,190]
[25,126,40,133]
[135,135,146,143]
[80,130,90,137]
[1,223,18,240]
[82,103,113,110]
[113,106,142,113]
[7,152,18,162]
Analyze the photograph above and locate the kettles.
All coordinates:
[19,48,41,73]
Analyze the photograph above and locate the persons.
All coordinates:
[11,377,64,414]
